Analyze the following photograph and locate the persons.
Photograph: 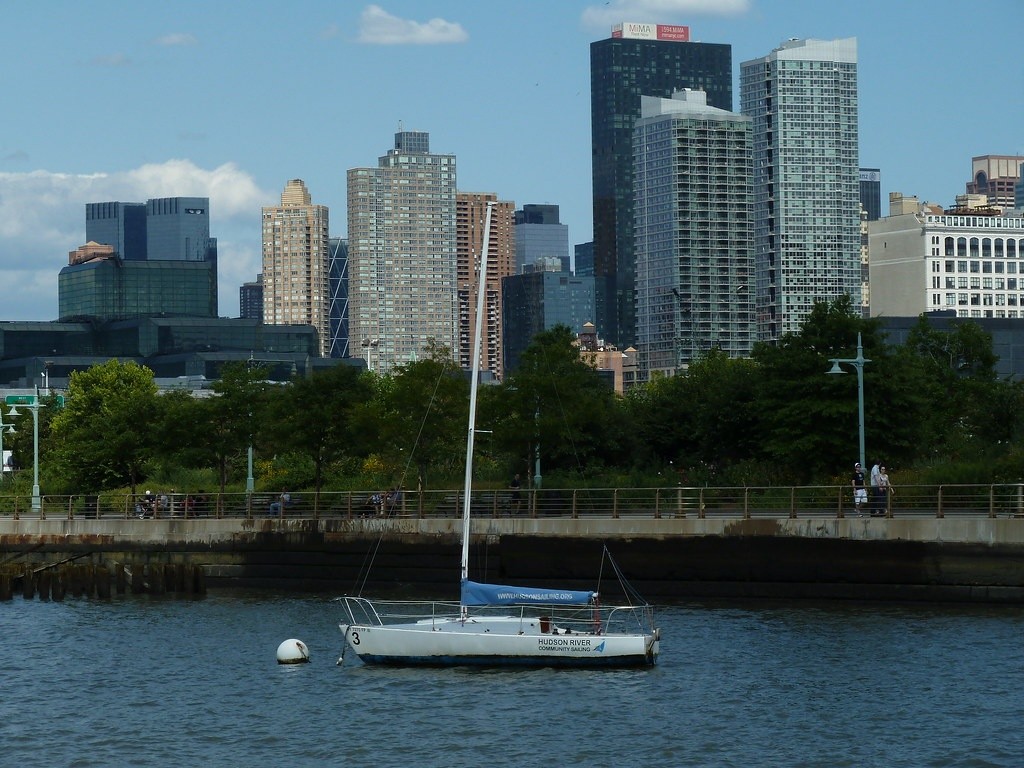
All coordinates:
[871,459,894,513]
[508,472,522,514]
[852,463,868,517]
[141,488,208,518]
[270,486,290,519]
[366,487,401,518]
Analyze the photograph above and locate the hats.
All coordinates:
[854,463,860,467]
[146,491,150,494]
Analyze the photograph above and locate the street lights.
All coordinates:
[42,360,55,395]
[245,356,298,513]
[4,385,43,513]
[0,409,20,482]
[360,338,380,370]
[824,331,872,478]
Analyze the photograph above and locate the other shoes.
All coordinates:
[854,509,860,513]
[857,515,863,517]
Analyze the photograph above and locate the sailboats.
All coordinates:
[331,200,665,670]
